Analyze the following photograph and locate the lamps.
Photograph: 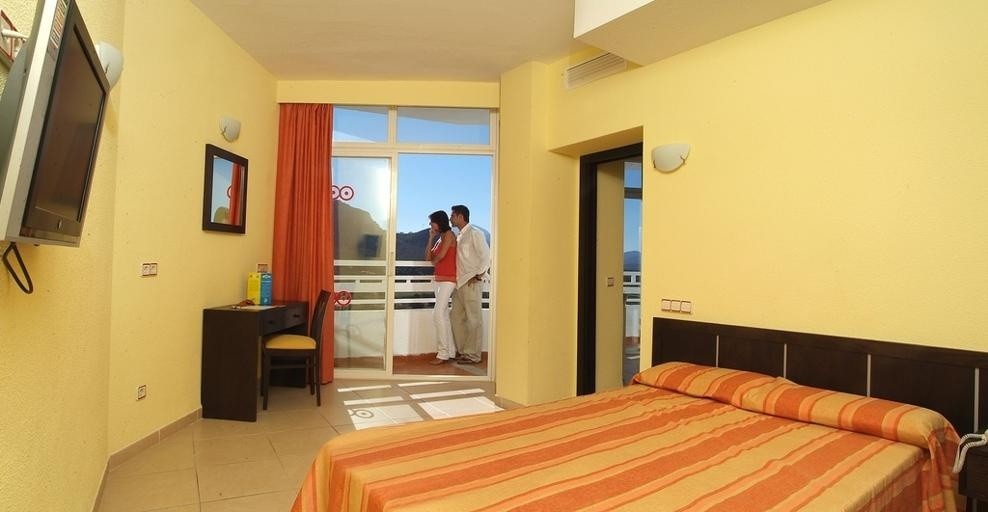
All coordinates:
[217,114,243,144]
[646,143,690,176]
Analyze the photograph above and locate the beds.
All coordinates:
[288,312,986,512]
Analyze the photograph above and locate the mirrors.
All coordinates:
[201,142,249,236]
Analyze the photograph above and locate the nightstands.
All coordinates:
[950,429,988,511]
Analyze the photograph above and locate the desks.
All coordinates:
[199,298,311,425]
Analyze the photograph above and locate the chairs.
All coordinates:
[258,287,332,412]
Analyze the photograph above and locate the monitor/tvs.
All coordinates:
[0,0,110,247]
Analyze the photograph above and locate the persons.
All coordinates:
[424,210,457,365]
[449,205,491,364]
